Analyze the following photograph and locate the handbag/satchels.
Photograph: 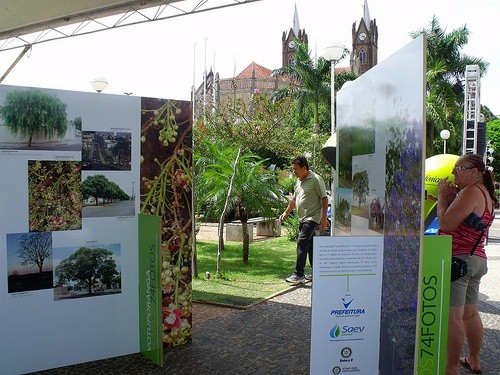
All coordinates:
[451,258,467,281]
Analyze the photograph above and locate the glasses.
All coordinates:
[451,165,473,170]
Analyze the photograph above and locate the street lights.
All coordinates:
[439,129,451,154]
[303,150,312,163]
[310,134,320,173]
[318,39,345,137]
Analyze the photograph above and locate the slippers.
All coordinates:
[459,356,481,375]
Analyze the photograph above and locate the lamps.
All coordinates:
[486,140,495,173]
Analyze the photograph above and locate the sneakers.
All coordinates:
[285,273,312,282]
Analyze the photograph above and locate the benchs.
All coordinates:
[226,216,281,242]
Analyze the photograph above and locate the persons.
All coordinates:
[279,156,328,283]
[437,154,498,375]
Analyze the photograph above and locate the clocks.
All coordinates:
[288,40,296,49]
[358,32,367,40]
